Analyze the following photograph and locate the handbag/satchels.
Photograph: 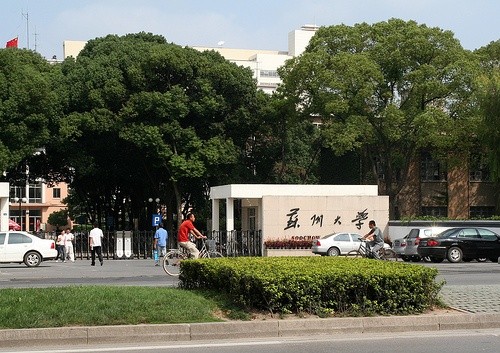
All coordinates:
[153,249,158,260]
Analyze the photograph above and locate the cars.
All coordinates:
[0,231,59,269]
[394,225,499,265]
[311,232,391,258]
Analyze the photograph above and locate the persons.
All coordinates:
[55,229,65,261]
[360,219,385,260]
[63,228,75,262]
[177,213,208,261]
[153,223,169,266]
[89,222,104,266]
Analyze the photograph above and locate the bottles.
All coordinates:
[91,243,93,250]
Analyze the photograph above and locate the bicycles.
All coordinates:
[345,236,398,265]
[162,236,225,277]
